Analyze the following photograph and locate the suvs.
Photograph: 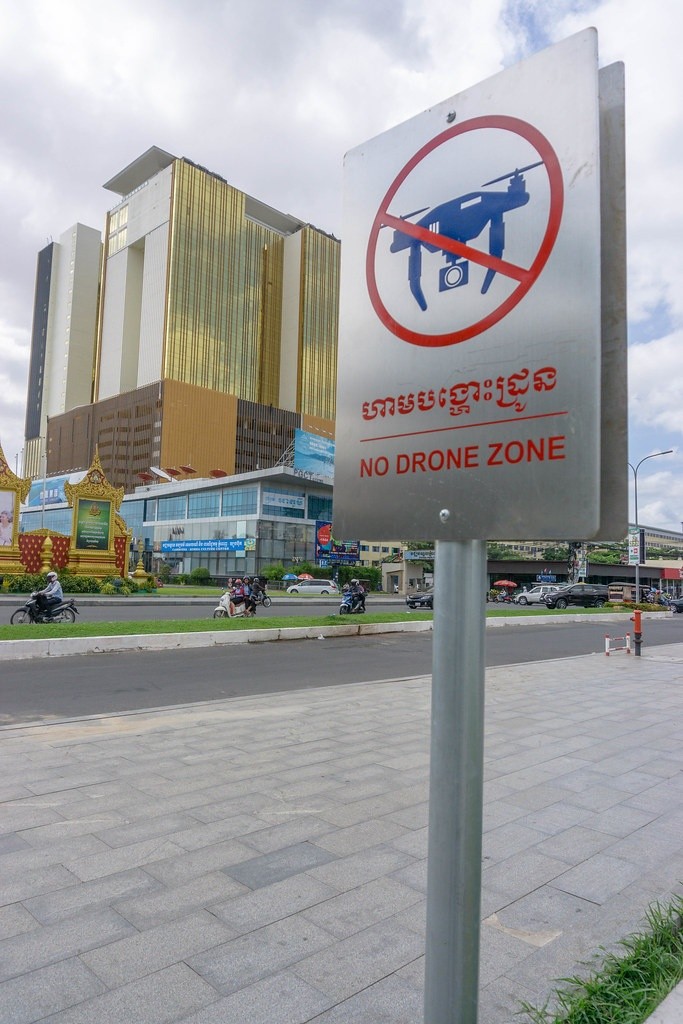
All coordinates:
[542,583,610,610]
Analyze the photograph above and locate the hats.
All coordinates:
[235,579,242,586]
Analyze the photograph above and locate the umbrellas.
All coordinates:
[494,580,517,589]
[282,573,312,581]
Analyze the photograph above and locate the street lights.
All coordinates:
[628,448,673,604]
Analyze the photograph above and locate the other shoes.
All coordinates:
[43,614,52,620]
[244,611,250,614]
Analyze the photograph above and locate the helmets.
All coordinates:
[656,590,660,594]
[47,572,58,581]
[351,579,358,586]
[253,578,259,583]
[243,575,250,580]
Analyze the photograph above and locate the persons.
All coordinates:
[646,590,672,607]
[228,576,266,617]
[35,572,63,621]
[342,579,366,613]
[500,586,528,604]
[0,511,13,546]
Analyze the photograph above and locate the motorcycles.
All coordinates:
[10,588,79,625]
[339,592,368,615]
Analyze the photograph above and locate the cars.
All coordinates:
[286,579,339,594]
[406,586,433,610]
[668,596,683,614]
[514,586,559,605]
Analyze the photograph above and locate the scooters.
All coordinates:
[654,596,671,606]
[213,586,271,618]
[494,594,513,604]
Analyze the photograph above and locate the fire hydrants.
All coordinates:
[630,610,643,655]
